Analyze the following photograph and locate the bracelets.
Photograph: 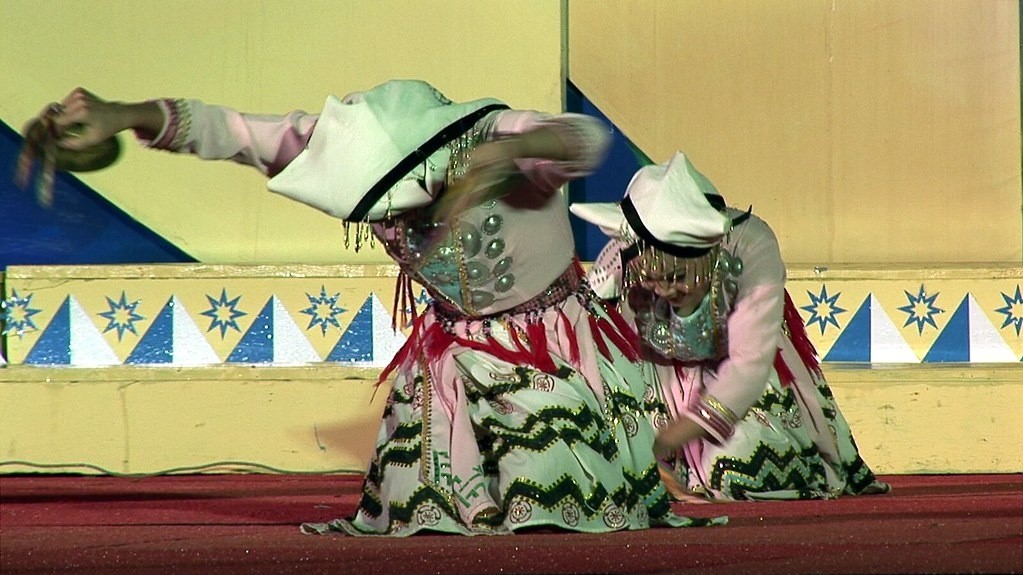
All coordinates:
[688,395,739,440]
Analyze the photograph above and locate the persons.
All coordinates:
[56,79,729,538]
[568,150,890,502]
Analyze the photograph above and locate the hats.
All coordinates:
[262,79,507,255]
[568,149,732,302]
